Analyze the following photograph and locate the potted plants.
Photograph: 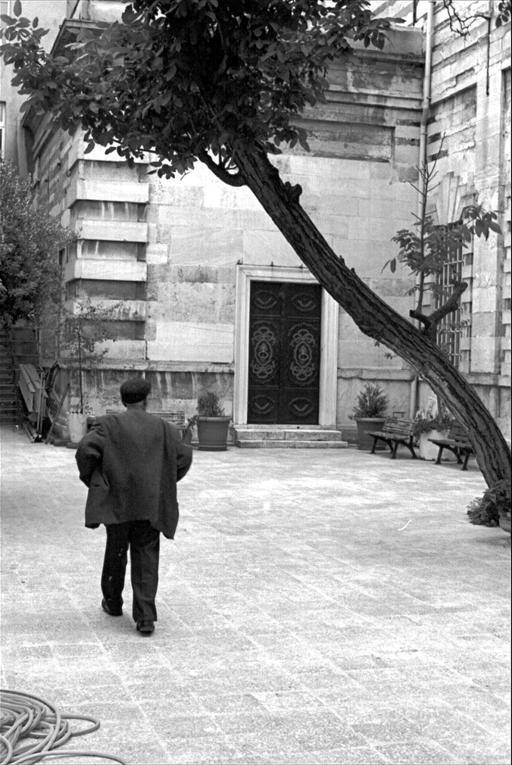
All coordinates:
[191,386,233,450]
[349,382,389,451]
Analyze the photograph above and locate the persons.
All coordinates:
[75,378,192,633]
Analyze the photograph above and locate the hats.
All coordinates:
[120,377,148,402]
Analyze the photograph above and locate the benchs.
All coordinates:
[367,415,478,473]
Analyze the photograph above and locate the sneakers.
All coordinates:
[102,596,123,616]
[136,620,154,632]
